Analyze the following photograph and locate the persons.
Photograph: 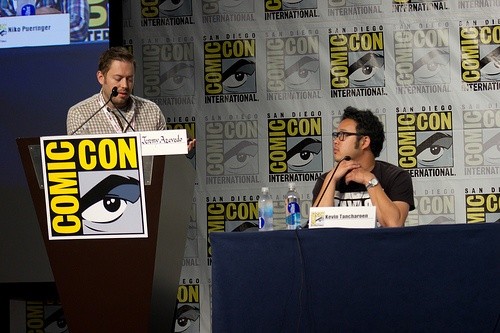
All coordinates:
[312,106,415,227]
[67,46,196,152]
[0,0,90,43]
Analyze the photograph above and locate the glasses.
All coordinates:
[332,131,364,141]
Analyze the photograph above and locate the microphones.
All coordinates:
[110,86,135,131]
[302,156,351,229]
[71,92,118,135]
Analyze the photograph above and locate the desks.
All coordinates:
[209,223,500,333]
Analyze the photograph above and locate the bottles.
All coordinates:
[258,187,273,231]
[284,182,300,229]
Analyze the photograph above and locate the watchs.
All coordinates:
[366,177,379,190]
